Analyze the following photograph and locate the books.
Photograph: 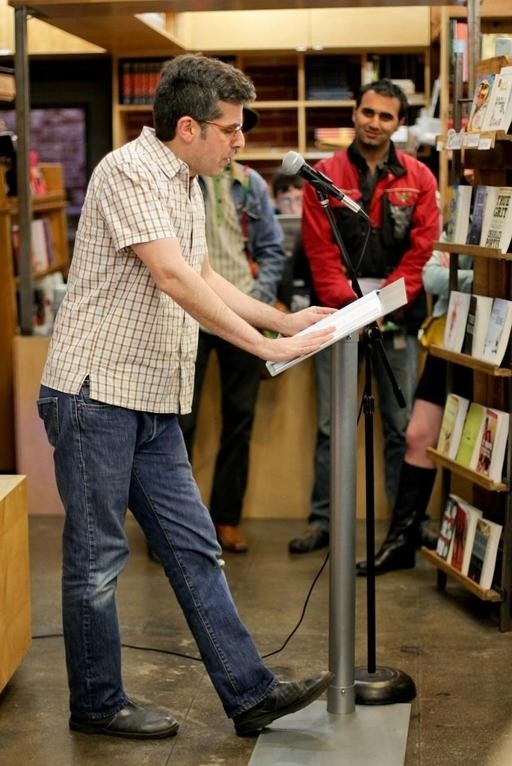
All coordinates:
[305,78,426,152]
[115,52,174,107]
[270,287,381,370]
[0,131,67,336]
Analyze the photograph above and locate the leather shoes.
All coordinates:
[215,523,248,554]
[232,670,335,736]
[69,700,179,738]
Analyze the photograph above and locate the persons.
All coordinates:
[288,78,423,556]
[144,97,287,564]
[36,52,338,740]
[354,128,476,576]
[268,169,323,311]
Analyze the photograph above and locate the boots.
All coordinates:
[356,461,437,577]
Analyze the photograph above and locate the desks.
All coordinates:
[0,472,40,701]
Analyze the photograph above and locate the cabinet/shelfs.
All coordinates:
[2,192,68,334]
[414,52,512,632]
[110,44,432,165]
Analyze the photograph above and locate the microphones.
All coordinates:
[280,149,369,220]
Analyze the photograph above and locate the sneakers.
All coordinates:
[289,524,329,553]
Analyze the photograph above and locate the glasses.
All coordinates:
[205,118,243,139]
